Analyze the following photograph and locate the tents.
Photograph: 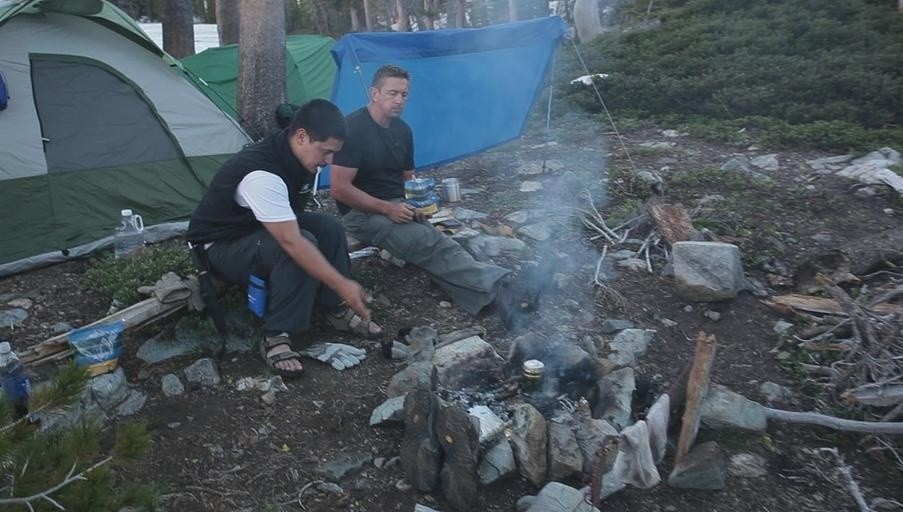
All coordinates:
[0,0,270,280]
[177,35,338,123]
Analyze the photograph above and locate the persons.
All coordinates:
[330,66,557,330]
[185,99,383,377]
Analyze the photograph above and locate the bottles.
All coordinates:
[0,340,32,403]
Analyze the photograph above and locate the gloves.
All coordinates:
[308,339,368,370]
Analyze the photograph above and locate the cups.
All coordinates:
[434,177,461,203]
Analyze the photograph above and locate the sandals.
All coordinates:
[325,307,384,339]
[260,333,304,378]
